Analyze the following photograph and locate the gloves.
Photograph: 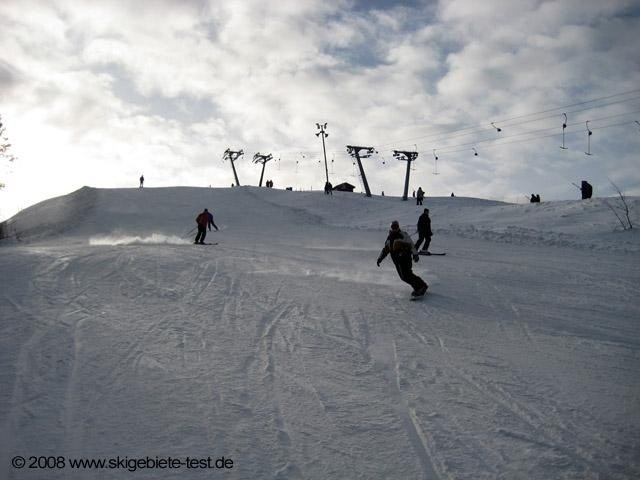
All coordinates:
[376,259,381,267]
[412,254,419,263]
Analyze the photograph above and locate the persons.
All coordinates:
[266,180,273,187]
[140,175,144,188]
[416,187,423,205]
[195,208,218,244]
[377,220,428,297]
[414,209,433,253]
[325,181,333,195]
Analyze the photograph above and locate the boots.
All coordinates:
[420,250,430,254]
[194,240,205,244]
[411,284,429,296]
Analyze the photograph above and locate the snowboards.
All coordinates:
[409,292,425,301]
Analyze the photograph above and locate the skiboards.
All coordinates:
[417,252,446,255]
[194,242,218,245]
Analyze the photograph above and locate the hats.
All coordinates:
[390,220,399,228]
